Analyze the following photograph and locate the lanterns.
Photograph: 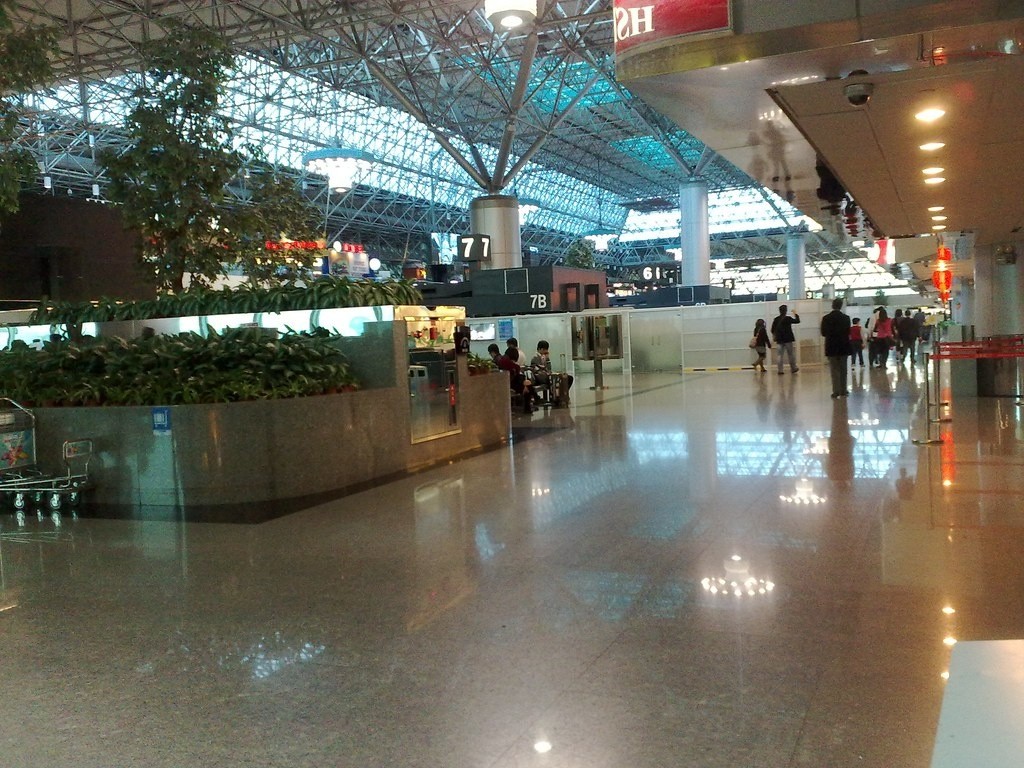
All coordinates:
[933,245,953,305]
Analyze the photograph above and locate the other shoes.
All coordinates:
[792,368,799,374]
[752,363,757,370]
[778,372,784,375]
[851,364,856,370]
[860,365,865,368]
[761,370,767,372]
[876,364,887,369]
[831,393,840,399]
[840,391,849,396]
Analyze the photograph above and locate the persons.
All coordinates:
[874,308,899,370]
[915,307,951,343]
[821,396,858,494]
[505,338,527,371]
[868,306,885,367]
[775,374,800,442]
[899,309,924,364]
[821,298,852,398]
[865,308,881,364]
[747,129,771,183]
[532,338,574,405]
[771,303,802,376]
[492,347,543,415]
[893,307,908,361]
[487,343,504,365]
[753,371,774,426]
[765,119,792,180]
[852,361,932,424]
[752,319,774,372]
[850,317,867,372]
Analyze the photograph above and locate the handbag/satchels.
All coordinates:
[749,337,758,348]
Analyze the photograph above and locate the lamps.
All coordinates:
[584,155,619,250]
[502,134,540,227]
[299,79,374,193]
[484,0,538,32]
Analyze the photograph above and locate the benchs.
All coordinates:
[511,364,552,414]
